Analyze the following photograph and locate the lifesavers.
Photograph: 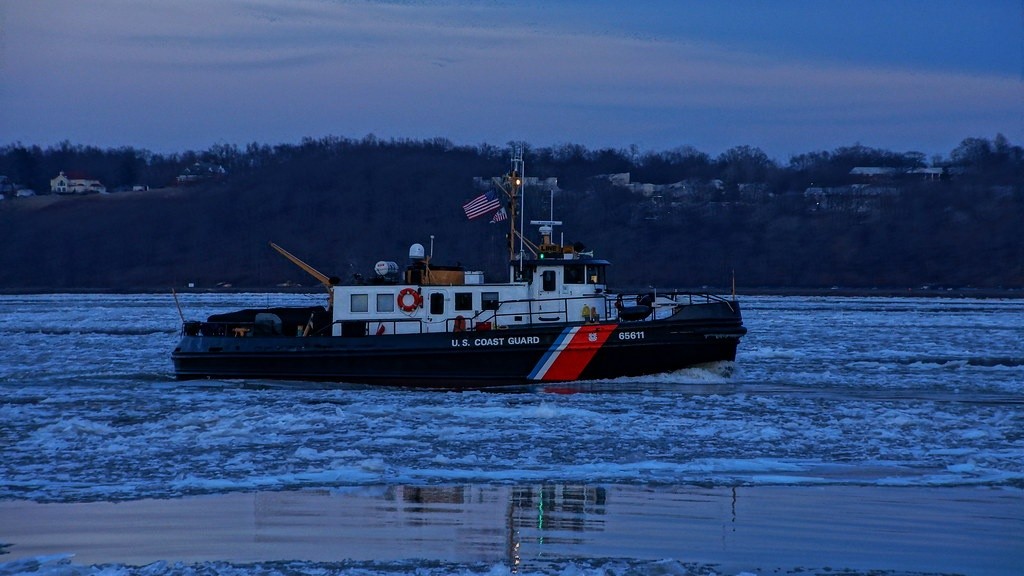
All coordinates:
[397,288,421,313]
[454,314,466,333]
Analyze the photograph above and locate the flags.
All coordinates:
[462,188,500,219]
[490,207,508,224]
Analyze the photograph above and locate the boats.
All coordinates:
[169,145,749,396]
